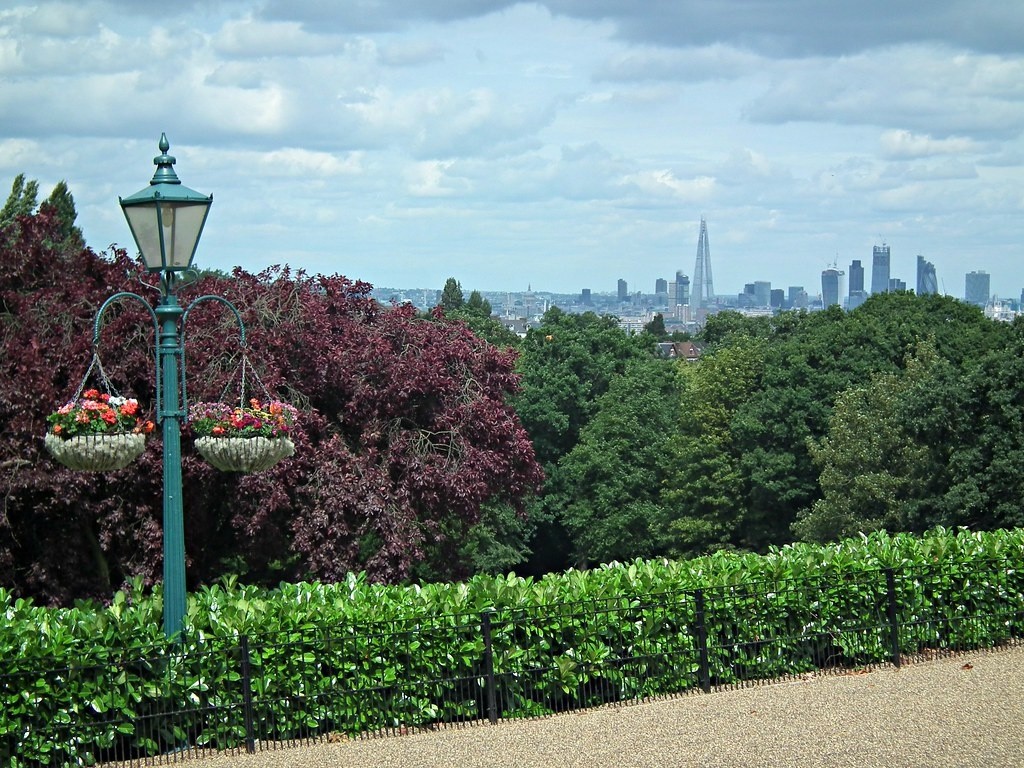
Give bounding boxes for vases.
[45,432,146,471]
[195,436,297,474]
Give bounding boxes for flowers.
[46,389,154,437]
[188,401,297,439]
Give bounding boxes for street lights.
[45,134,297,647]
